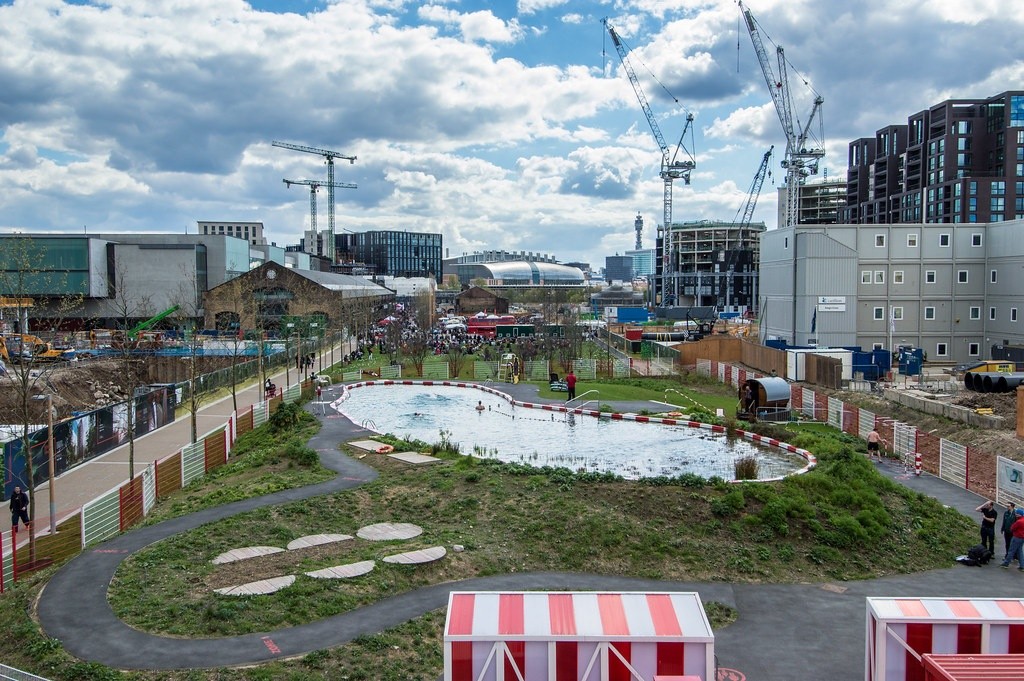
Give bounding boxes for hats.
[1015,508,1024,516]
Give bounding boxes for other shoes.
[990,555,995,559]
[1017,567,1024,571]
[1000,563,1009,568]
[879,460,882,463]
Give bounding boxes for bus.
[466,325,496,337]
[468,315,518,325]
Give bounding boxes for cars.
[519,315,552,325]
[437,311,468,333]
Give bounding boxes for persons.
[741,386,752,410]
[975,501,1024,572]
[566,371,577,401]
[265,378,272,395]
[866,427,883,463]
[9,486,30,535]
[309,371,316,384]
[294,308,607,374]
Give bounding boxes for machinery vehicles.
[88,302,180,346]
[0,297,64,362]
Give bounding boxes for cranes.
[282,178,358,231]
[274,141,357,262]
[733,0,827,227]
[598,14,699,308]
